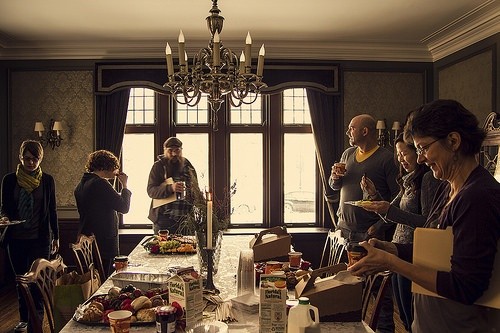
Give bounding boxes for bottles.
[155,305,177,333]
[176,181,186,200]
[286,297,319,333]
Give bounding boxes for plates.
[344,200,386,207]
[0,220,20,226]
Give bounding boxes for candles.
[207,192,212,249]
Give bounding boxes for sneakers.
[15,322,28,332]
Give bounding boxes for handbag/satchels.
[53,270,100,333]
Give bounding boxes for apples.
[108,288,119,298]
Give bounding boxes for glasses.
[415,137,443,155]
[21,156,39,162]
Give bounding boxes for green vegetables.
[159,241,181,254]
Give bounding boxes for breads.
[177,244,193,251]
[295,269,310,277]
[282,262,289,271]
[82,295,163,322]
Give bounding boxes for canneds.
[155,305,177,333]
[265,261,280,274]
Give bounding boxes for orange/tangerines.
[274,280,286,288]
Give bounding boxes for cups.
[287,252,302,268]
[107,310,132,333]
[114,255,128,272]
[334,163,346,176]
[158,230,168,241]
[347,241,368,266]
[238,249,254,271]
[265,261,280,274]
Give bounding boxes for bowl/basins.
[109,272,170,291]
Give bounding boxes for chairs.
[318,229,391,332]
[15,254,68,333]
[69,233,106,281]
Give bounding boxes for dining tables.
[58,234,374,333]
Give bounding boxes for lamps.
[376,120,400,149]
[33,120,63,151]
[162,0,268,131]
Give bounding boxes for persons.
[360,129,441,333]
[73,150,132,282]
[328,114,404,333]
[147,136,205,240]
[340,96,500,333]
[0,138,60,332]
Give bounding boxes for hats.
[164,137,182,147]
